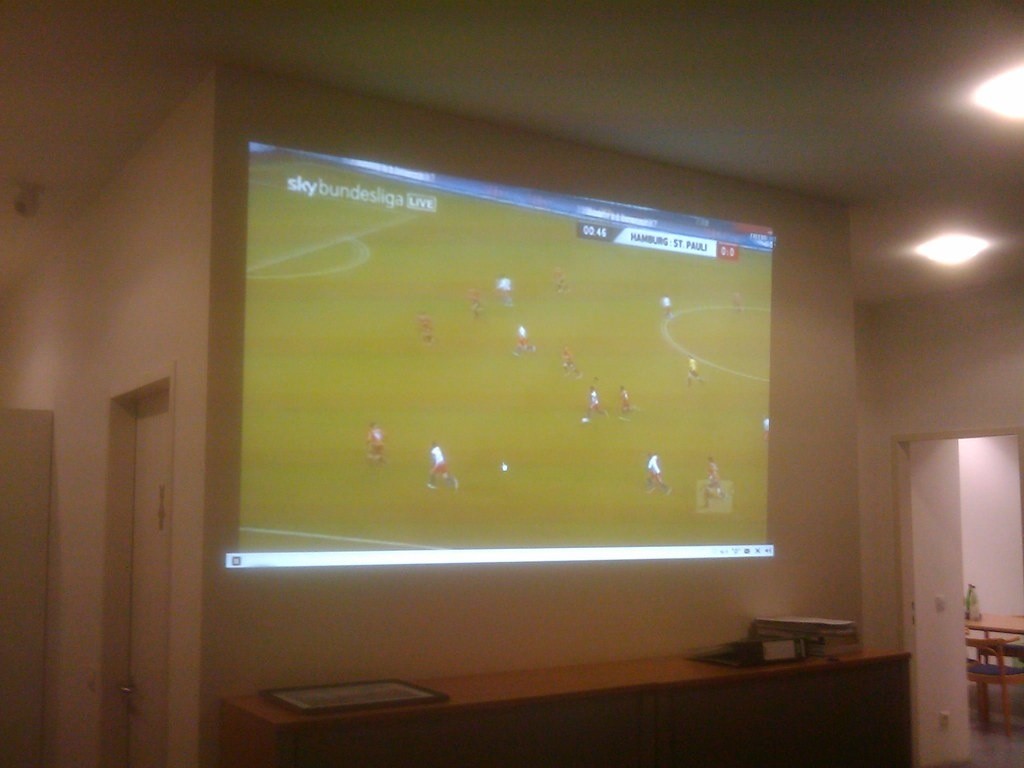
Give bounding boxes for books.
[756,614,861,656]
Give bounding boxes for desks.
[219,647,912,768]
[967,613,1024,664]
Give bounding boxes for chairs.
[966,636,1024,737]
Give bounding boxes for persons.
[687,356,702,385]
[510,324,537,358]
[562,346,581,379]
[581,386,608,423]
[365,422,387,463]
[700,458,726,510]
[733,292,741,308]
[763,416,769,440]
[551,266,573,293]
[493,275,513,307]
[644,450,672,496]
[661,295,674,320]
[618,386,631,419]
[464,288,481,318]
[426,444,460,491]
[418,310,434,345]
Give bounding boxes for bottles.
[966,584,980,622]
